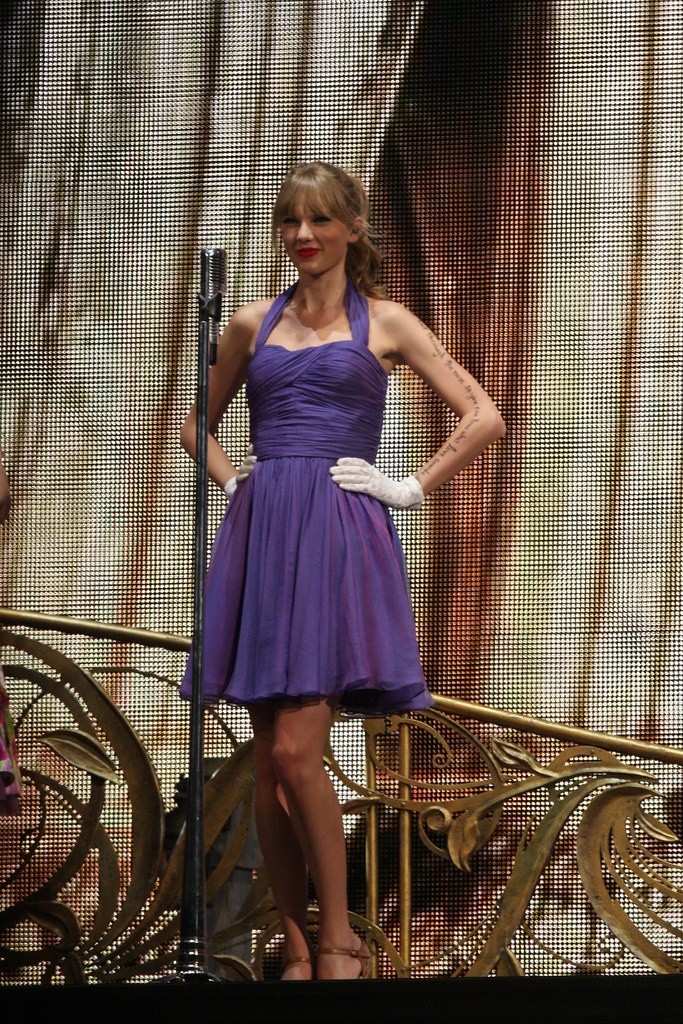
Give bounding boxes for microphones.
[199,248,228,365]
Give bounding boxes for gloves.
[330,456,425,510]
[225,445,258,499]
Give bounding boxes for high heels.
[317,933,373,980]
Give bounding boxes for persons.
[180,161,506,979]
